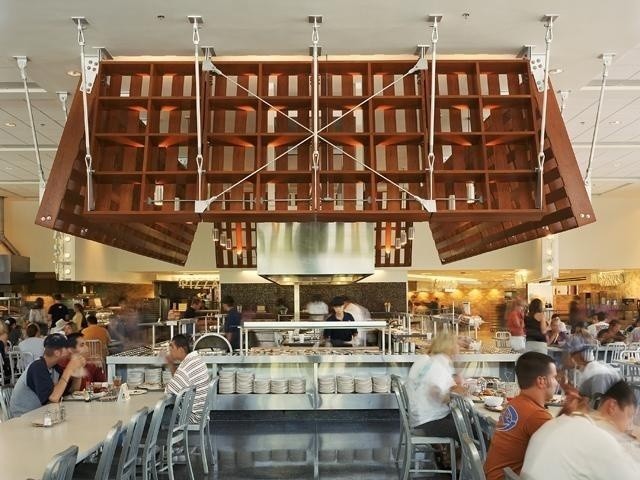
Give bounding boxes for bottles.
[44,405,65,426]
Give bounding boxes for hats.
[43,333,67,346]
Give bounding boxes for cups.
[426,332,432,339]
[288,331,294,341]
[112,376,123,387]
[392,341,416,352]
[467,377,518,401]
[299,334,304,343]
[84,382,114,401]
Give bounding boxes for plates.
[139,386,162,390]
[218,447,392,467]
[484,405,503,411]
[129,389,148,395]
[217,370,390,394]
[127,367,172,386]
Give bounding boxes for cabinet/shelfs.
[86,57,547,221]
[198,409,390,473]
[418,77,598,267]
[108,339,542,407]
[373,190,414,268]
[35,71,205,267]
[209,192,258,268]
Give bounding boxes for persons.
[180,298,207,342]
[323,295,371,348]
[482,351,580,480]
[141,334,211,436]
[404,334,477,480]
[506,294,639,415]
[408,294,438,315]
[1,293,128,418]
[220,296,241,352]
[520,380,638,480]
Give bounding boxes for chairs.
[448,312,638,480]
[2,382,218,479]
[390,379,459,477]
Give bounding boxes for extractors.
[256,221,375,286]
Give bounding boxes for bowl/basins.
[484,396,503,407]
[209,326,221,332]
